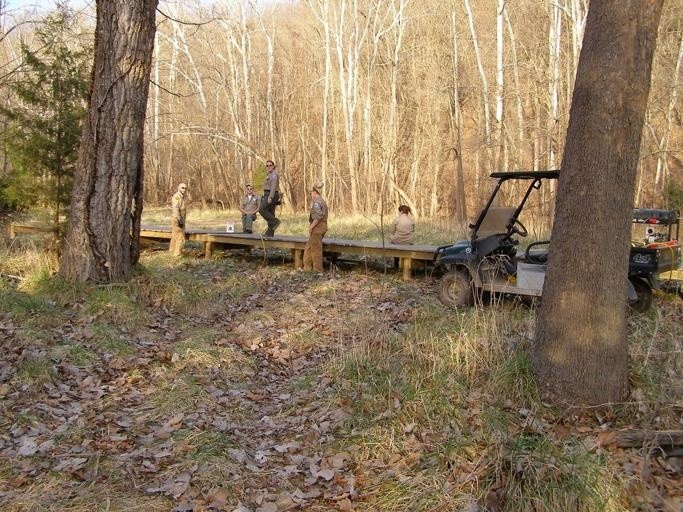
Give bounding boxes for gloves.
[178,219,183,228]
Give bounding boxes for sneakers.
[261,218,281,237]
[395,258,399,268]
[313,272,323,276]
[244,230,252,234]
[173,254,185,258]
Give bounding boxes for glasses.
[247,188,252,190]
[310,193,312,195]
[266,164,272,167]
[182,187,187,189]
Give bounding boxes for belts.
[264,190,278,192]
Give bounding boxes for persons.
[240,183,260,253]
[298,186,329,276]
[388,205,415,268]
[259,160,282,237]
[169,183,192,257]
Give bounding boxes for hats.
[246,183,254,188]
[313,185,321,193]
